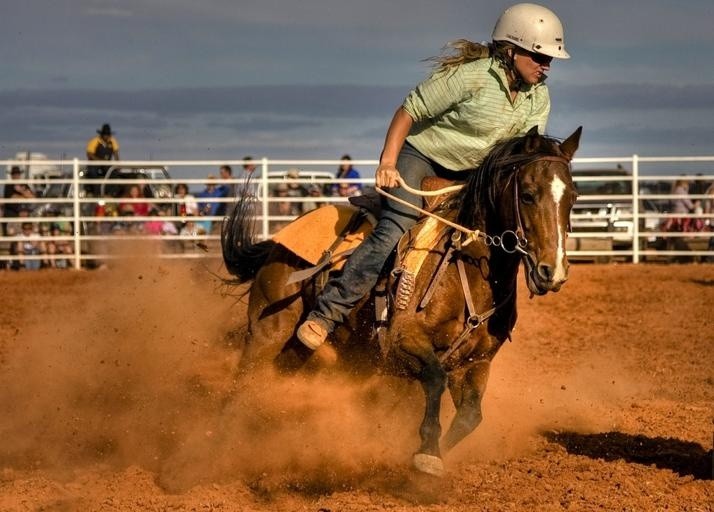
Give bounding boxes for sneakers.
[296,317,329,352]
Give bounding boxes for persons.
[79,122,120,197]
[293,1,570,350]
[671,175,700,217]
[270,154,362,234]
[197,157,256,249]
[1,166,199,272]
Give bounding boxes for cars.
[569,163,671,261]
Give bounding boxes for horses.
[202,123,583,479]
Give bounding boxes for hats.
[96,123,115,136]
[6,167,24,175]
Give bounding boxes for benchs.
[565,237,626,265]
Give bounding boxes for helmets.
[491,1,572,60]
